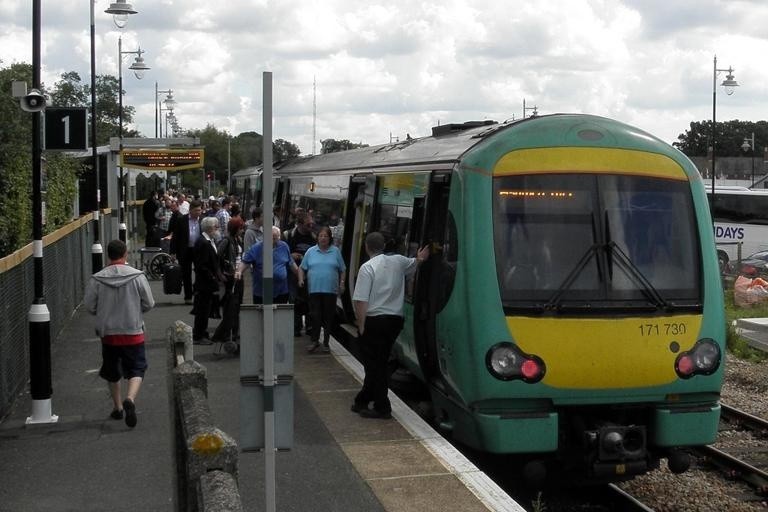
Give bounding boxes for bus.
[698,180,767,272]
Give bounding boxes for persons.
[296,225,348,355]
[348,231,431,420]
[142,184,346,346]
[84,239,156,429]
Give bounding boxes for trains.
[230,114,726,482]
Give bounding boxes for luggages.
[163,261,183,295]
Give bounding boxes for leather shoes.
[350,402,368,412]
[361,407,391,419]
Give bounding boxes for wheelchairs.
[146,241,176,281]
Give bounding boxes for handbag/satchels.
[189,294,221,320]
[288,295,310,314]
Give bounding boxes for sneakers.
[322,344,331,352]
[193,336,213,345]
[123,398,137,428]
[305,341,320,352]
[185,300,193,304]
[110,408,123,420]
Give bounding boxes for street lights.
[117,42,150,139]
[89,0,139,211]
[711,56,739,216]
[156,87,184,139]
[739,132,756,189]
